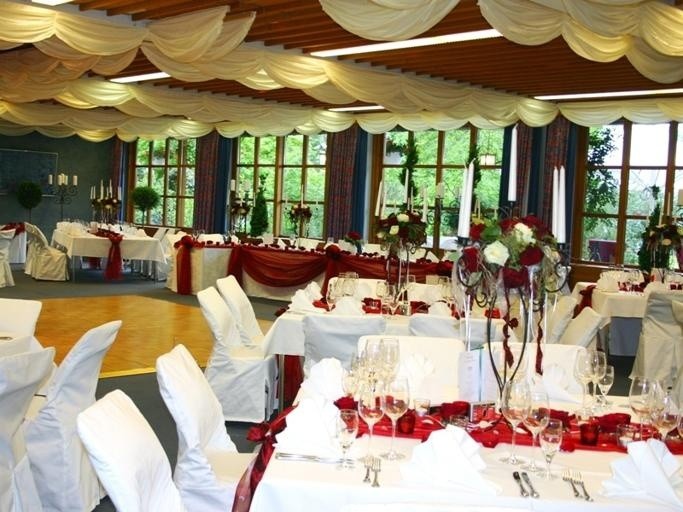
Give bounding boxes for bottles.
[224,230,231,244]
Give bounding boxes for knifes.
[513,472,529,498]
[275,450,353,464]
[521,472,539,497]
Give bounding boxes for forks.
[562,469,580,498]
[370,457,381,487]
[573,471,593,500]
[363,456,373,482]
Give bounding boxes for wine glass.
[500,380,563,477]
[450,414,468,431]
[662,266,683,290]
[607,262,641,296]
[327,272,360,315]
[375,274,416,315]
[193,230,198,242]
[573,348,615,415]
[198,229,205,241]
[339,335,429,460]
[649,389,678,446]
[629,376,656,443]
[437,274,468,317]
[677,408,683,438]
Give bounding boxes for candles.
[645,188,683,227]
[225,179,258,204]
[455,123,565,244]
[87,180,121,201]
[376,170,429,221]
[48,172,77,187]
[283,184,316,208]
